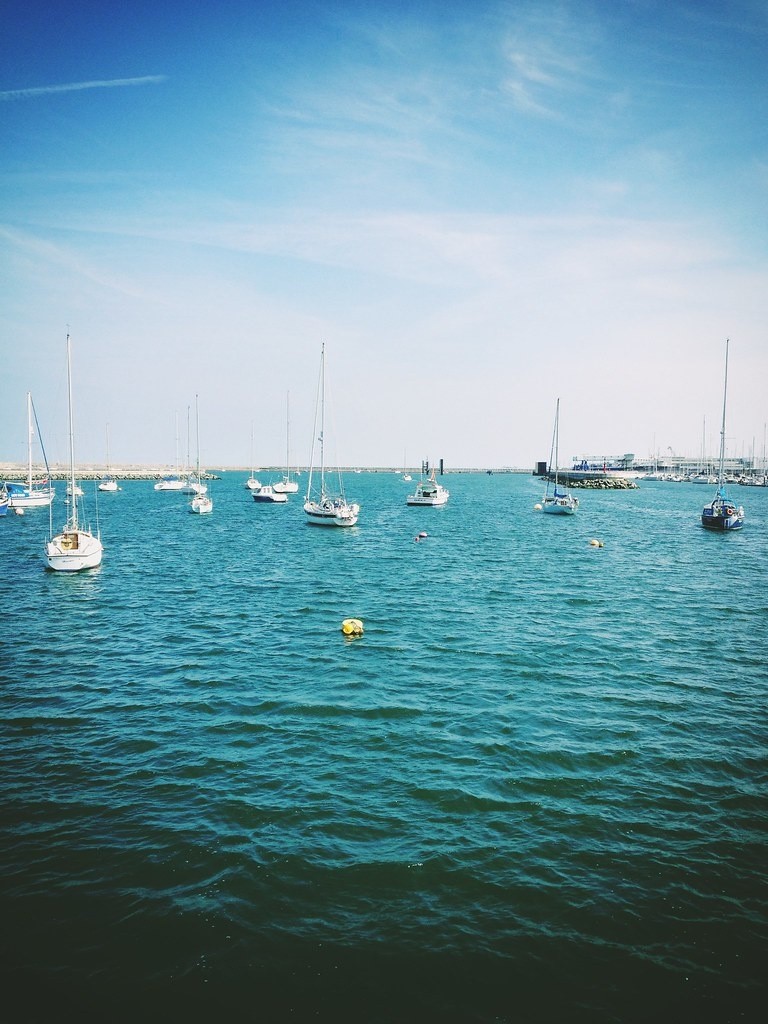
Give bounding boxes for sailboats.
[0,390,55,518]
[41,322,105,572]
[303,342,361,526]
[153,386,213,515]
[245,389,299,503]
[541,397,579,515]
[641,413,768,487]
[407,460,450,505]
[702,336,745,531]
[98,422,123,491]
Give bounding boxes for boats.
[66,474,85,497]
